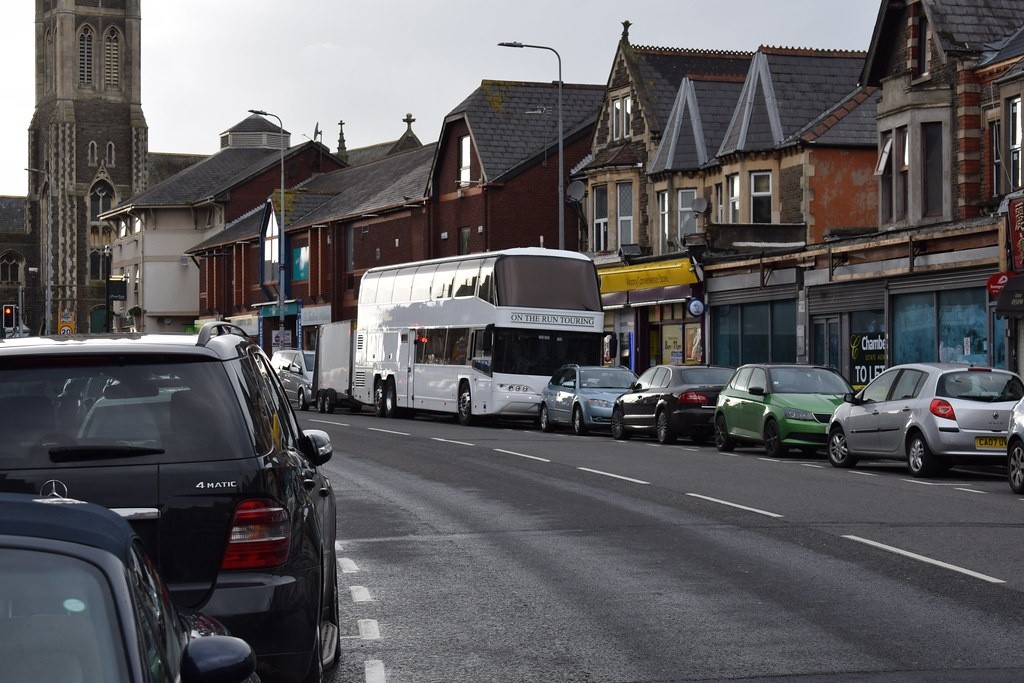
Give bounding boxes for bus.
[352,247,617,426]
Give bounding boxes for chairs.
[161,386,220,454]
[0,396,56,456]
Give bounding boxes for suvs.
[0,322,341,683]
[270,348,316,411]
[537,364,639,435]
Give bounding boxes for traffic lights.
[3,305,15,328]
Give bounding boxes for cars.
[610,364,735,444]
[1007,394,1024,495]
[827,362,1024,478]
[0,490,260,683]
[55,373,192,442]
[713,363,858,457]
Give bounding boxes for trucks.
[311,319,356,414]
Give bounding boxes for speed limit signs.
[60,325,73,335]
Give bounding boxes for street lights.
[497,42,563,249]
[103,245,110,333]
[25,168,52,335]
[248,109,285,350]
